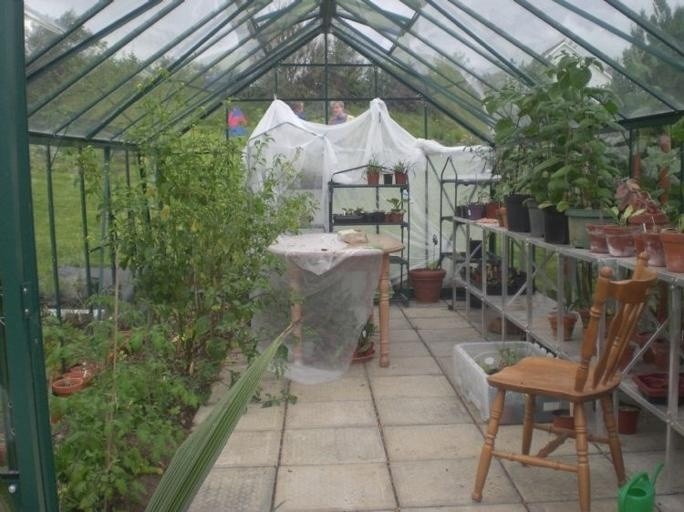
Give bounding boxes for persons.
[328,100,355,125]
[291,99,306,121]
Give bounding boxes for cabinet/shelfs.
[446,214,683,494]
[328,164,410,304]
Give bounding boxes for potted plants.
[408,235,446,305]
[447,53,683,433]
[39,312,88,400]
[334,155,418,226]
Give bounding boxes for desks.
[269,231,406,371]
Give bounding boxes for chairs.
[471,248,658,512]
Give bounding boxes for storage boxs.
[454,338,568,427]
